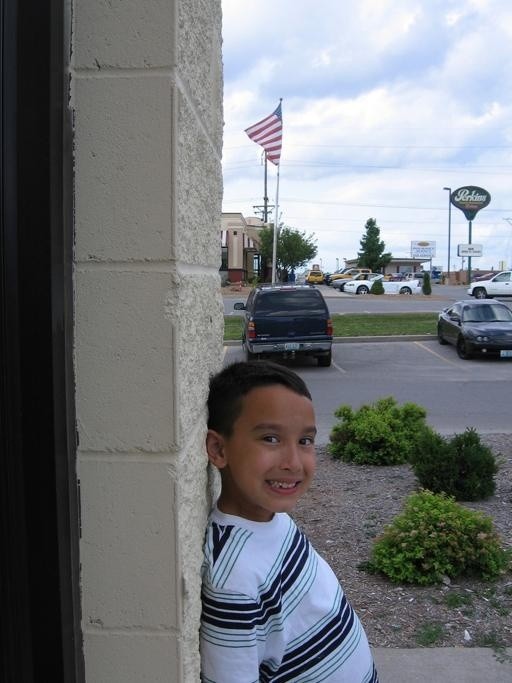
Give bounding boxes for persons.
[196,360,380,682]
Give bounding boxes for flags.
[243,96,284,167]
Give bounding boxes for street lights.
[336,258,339,270]
[443,187,451,285]
[320,258,322,270]
[252,150,280,282]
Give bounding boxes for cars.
[234,282,333,367]
[437,270,512,360]
[304,269,442,296]
